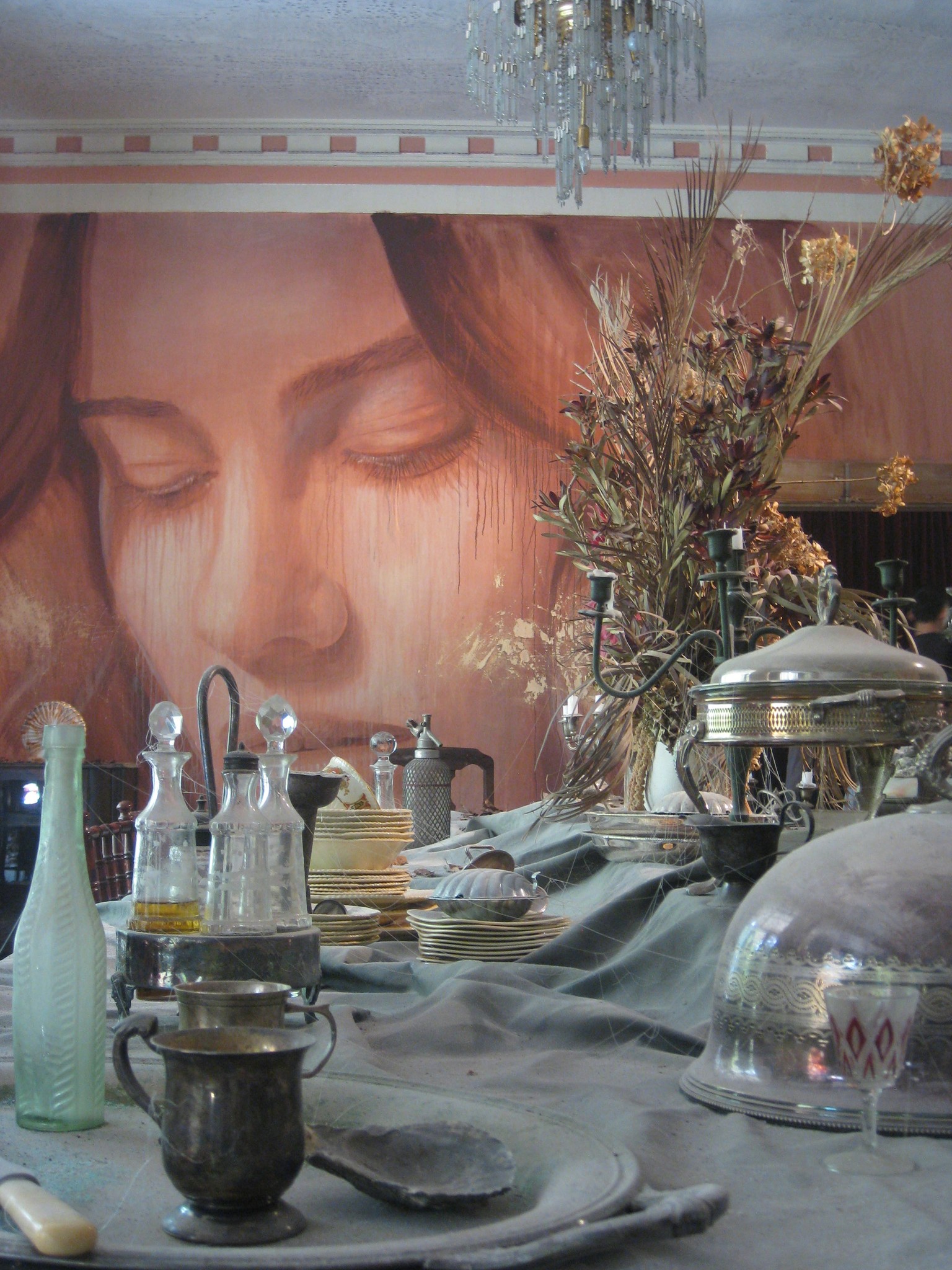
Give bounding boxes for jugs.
[684,800,816,906]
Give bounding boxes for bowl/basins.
[580,812,775,866]
[319,757,381,811]
[309,809,415,870]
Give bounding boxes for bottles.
[370,731,397,809]
[255,694,312,931]
[201,743,277,936]
[127,701,202,934]
[12,725,106,1133]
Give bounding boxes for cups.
[111,1011,318,1245]
[173,981,336,1079]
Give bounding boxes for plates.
[1,1055,640,1268]
[405,909,571,963]
[309,903,381,946]
[307,869,412,905]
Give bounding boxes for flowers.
[512,114,952,834]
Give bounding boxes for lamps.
[466,0,706,211]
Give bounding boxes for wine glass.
[823,984,920,1178]
